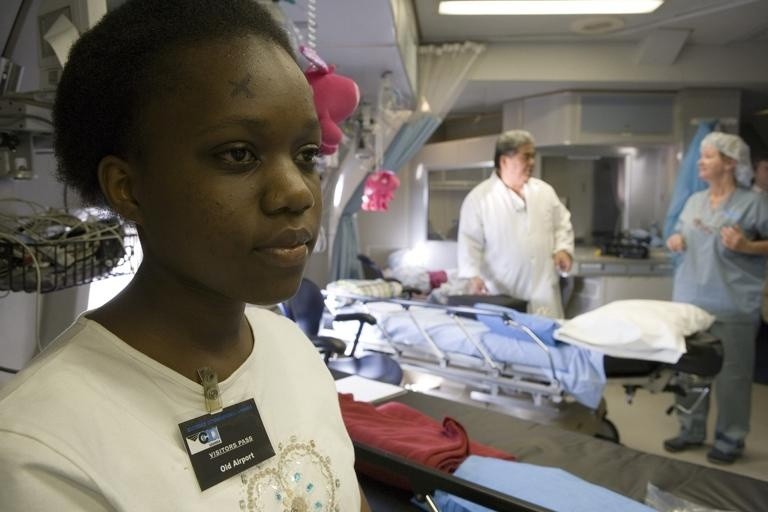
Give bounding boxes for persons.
[1,3,375,511]
[663,129,768,464]
[748,157,768,388]
[456,128,575,319]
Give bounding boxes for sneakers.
[707,448,741,466]
[664,436,703,452]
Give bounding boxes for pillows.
[549,294,718,369]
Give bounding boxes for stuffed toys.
[359,167,401,213]
[301,65,362,152]
